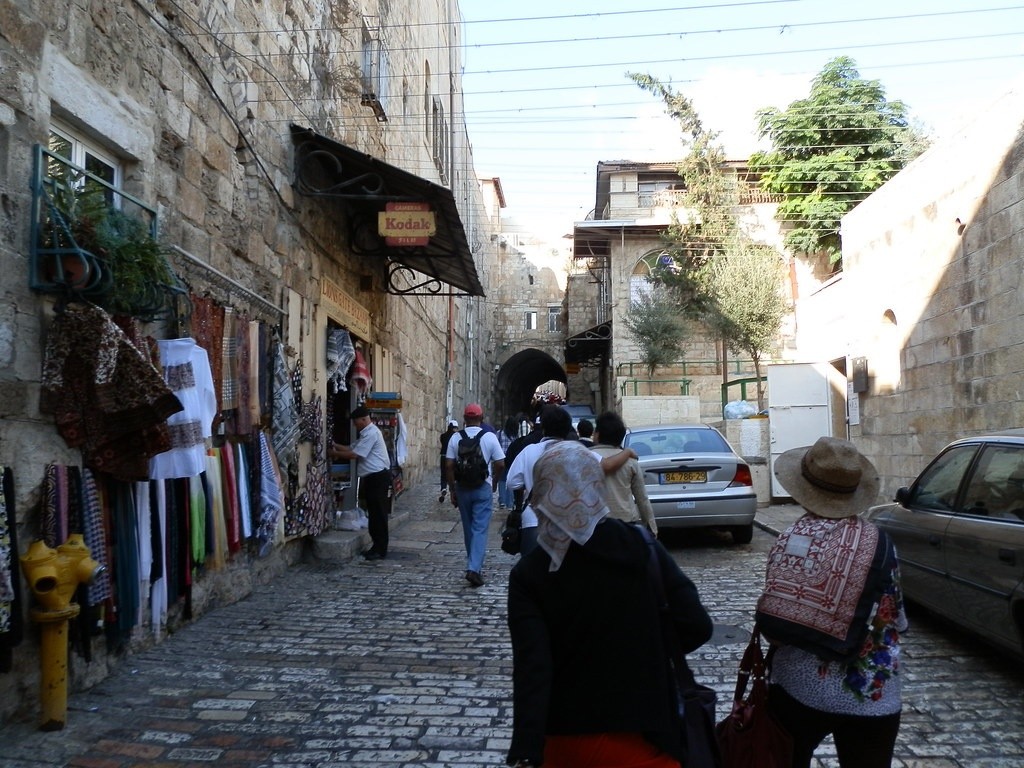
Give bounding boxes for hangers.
[150,316,188,337]
[57,279,91,313]
[23,422,77,466]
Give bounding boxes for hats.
[349,407,368,419]
[464,403,482,418]
[449,420,458,426]
[774,436,881,518]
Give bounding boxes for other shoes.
[500,503,506,509]
[365,545,386,560]
[439,488,447,502]
[466,569,484,586]
[507,507,513,511]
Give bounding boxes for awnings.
[291,123,486,297]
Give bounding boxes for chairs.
[684,441,704,452]
[1000,470,1024,520]
[630,442,653,456]
[330,457,353,490]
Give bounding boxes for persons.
[446,405,506,586]
[482,411,544,511]
[505,405,639,559]
[577,419,594,447]
[328,408,389,561]
[507,440,714,768]
[756,436,908,768]
[439,420,459,503]
[588,411,658,540]
[528,389,567,421]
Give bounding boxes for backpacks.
[453,429,490,489]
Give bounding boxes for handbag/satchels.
[501,508,523,554]
[674,683,718,768]
[713,622,794,768]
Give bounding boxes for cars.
[567,404,598,439]
[859,426,1023,662]
[619,427,757,545]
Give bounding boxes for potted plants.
[36,172,190,320]
